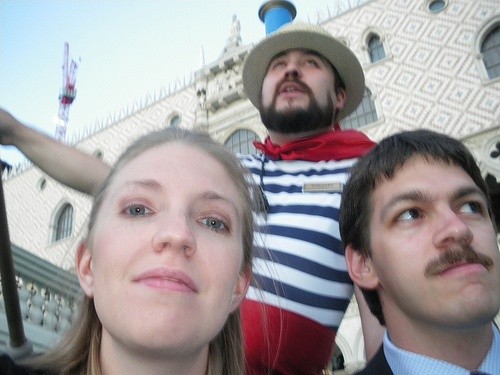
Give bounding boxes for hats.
[241,21,365,121]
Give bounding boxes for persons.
[0,127,254,375]
[1,24,386,375]
[337,127,499,375]
